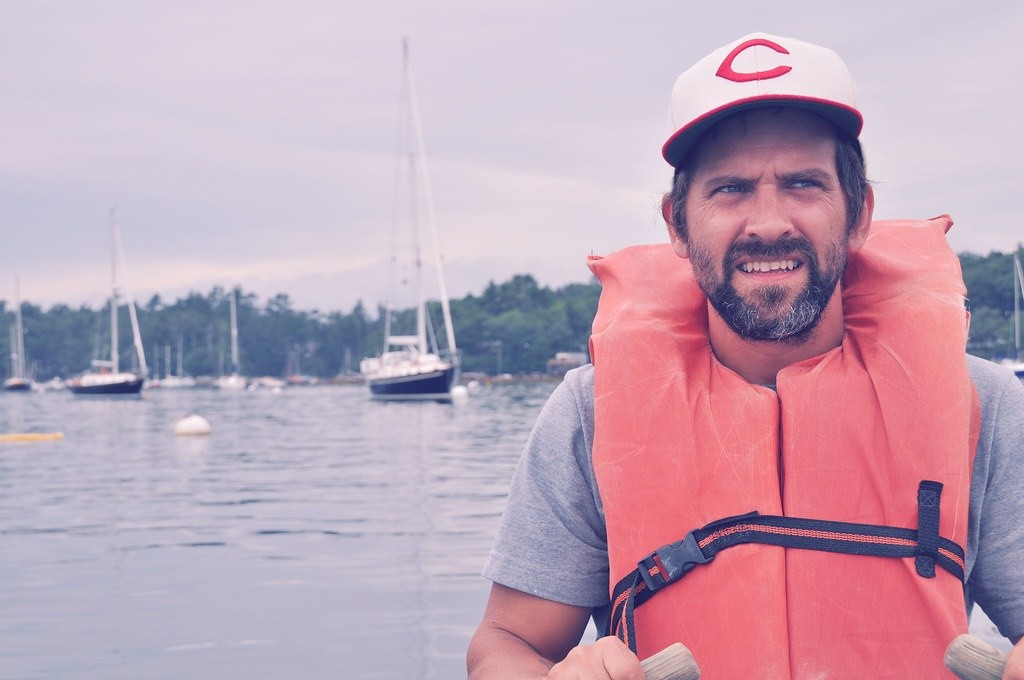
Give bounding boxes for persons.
[466,30,1024,680]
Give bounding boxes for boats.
[331,343,361,385]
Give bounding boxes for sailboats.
[1,275,68,392]
[359,37,462,403]
[142,286,318,389]
[67,207,152,394]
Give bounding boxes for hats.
[662,32,864,168]
